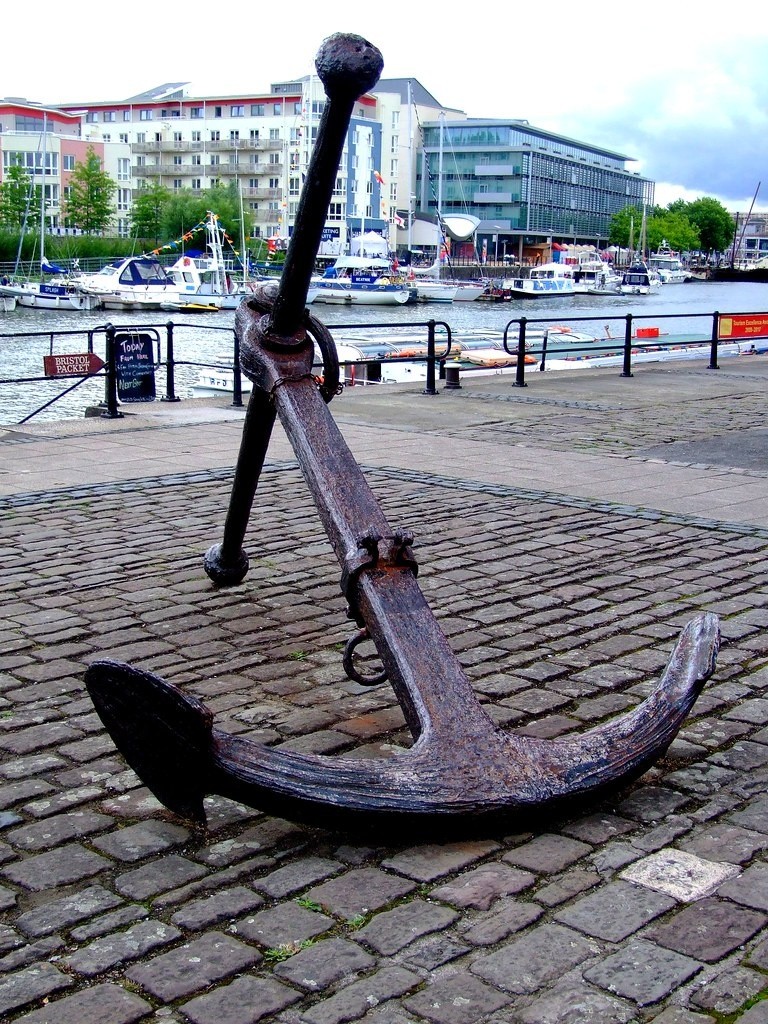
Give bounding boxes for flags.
[396,215,405,227]
[374,170,384,185]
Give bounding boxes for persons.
[484,284,511,296]
[535,250,542,266]
[2,274,12,286]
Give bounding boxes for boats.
[574,260,622,292]
[158,301,219,313]
[683,262,712,282]
[620,201,663,295]
[188,349,255,398]
[51,256,182,312]
[589,287,623,296]
[650,241,688,284]
[501,263,577,296]
[479,288,511,302]
[163,251,255,309]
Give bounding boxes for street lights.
[493,226,502,262]
[595,234,601,260]
[548,229,554,262]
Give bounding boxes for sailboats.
[228,61,486,305]
[0,112,102,310]
